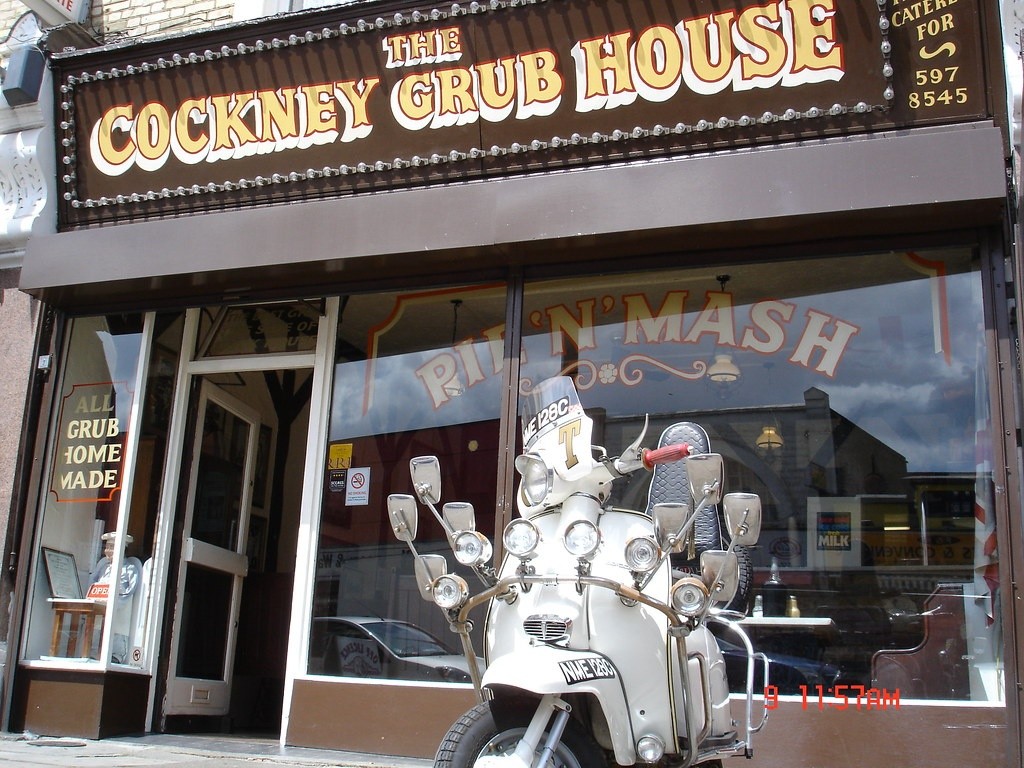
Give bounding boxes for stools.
[46,598,106,660]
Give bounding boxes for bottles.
[788,516,801,567]
[752,595,763,618]
[785,599,800,617]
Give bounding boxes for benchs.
[870,582,974,700]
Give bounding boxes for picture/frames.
[233,510,268,566]
[320,448,357,531]
[41,546,84,599]
[229,417,273,509]
[141,340,177,439]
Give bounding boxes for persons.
[79,532,139,664]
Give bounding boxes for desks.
[735,616,840,638]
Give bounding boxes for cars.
[307,614,489,685]
[712,631,872,700]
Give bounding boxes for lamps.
[702,274,745,402]
[753,409,786,460]
[442,298,466,400]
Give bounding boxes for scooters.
[385,377,770,768]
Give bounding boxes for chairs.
[646,422,724,567]
